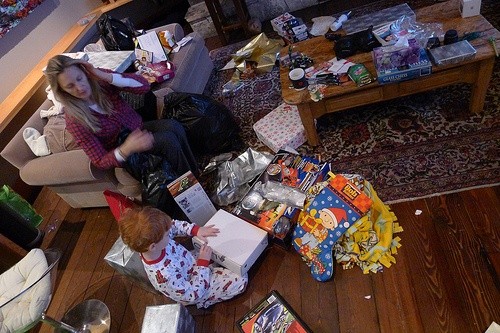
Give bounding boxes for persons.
[119,207,248,310]
[47,55,198,210]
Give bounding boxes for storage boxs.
[104,235,160,296]
[140,303,195,333]
[428,39,476,67]
[191,209,268,276]
[375,47,432,84]
[232,149,330,252]
[253,102,317,154]
[459,0,481,18]
[270,12,309,42]
[167,170,217,227]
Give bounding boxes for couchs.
[0,22,213,208]
[184,0,328,39]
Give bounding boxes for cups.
[267,162,283,182]
[289,68,308,91]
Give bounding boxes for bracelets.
[117,147,128,161]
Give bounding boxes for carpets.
[202,0,500,204]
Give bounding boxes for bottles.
[427,37,440,50]
[444,29,458,45]
[330,11,353,32]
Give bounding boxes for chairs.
[0,247,111,333]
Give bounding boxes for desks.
[280,0,500,146]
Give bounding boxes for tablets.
[236,288,314,333]
